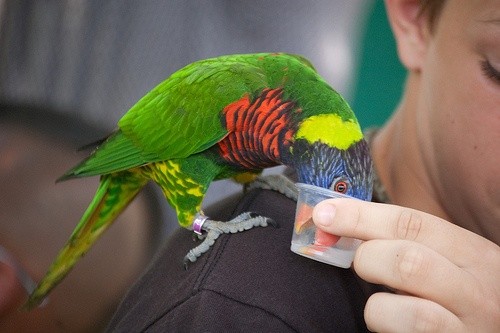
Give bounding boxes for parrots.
[21,52,371,311]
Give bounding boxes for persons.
[107,1,500,333]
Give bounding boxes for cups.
[290,182,362,269]
